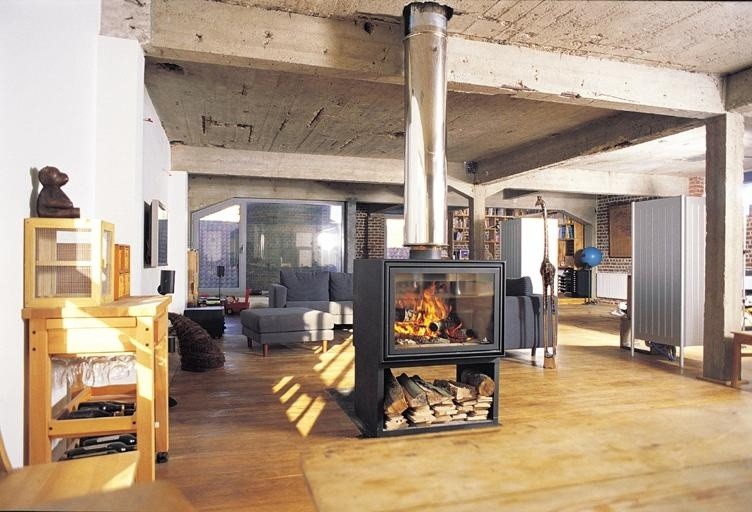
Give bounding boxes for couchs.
[268,282,558,357]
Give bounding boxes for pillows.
[329,271,353,301]
[280,269,330,301]
[506,276,533,296]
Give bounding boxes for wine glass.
[47,355,137,391]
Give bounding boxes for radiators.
[596,272,631,300]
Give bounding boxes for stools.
[239,306,335,357]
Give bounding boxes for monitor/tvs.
[151,199,168,267]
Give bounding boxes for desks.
[730,331,752,387]
[22,293,173,484]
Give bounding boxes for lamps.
[217,264,225,306]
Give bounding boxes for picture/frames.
[607,203,631,258]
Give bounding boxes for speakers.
[217,266,224,277]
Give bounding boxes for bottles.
[59,401,137,462]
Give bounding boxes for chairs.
[0,427,140,512]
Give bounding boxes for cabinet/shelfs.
[24,216,115,308]
[188,251,199,307]
[185,305,226,340]
[56,242,130,301]
[448,210,584,268]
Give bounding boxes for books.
[197,296,221,307]
